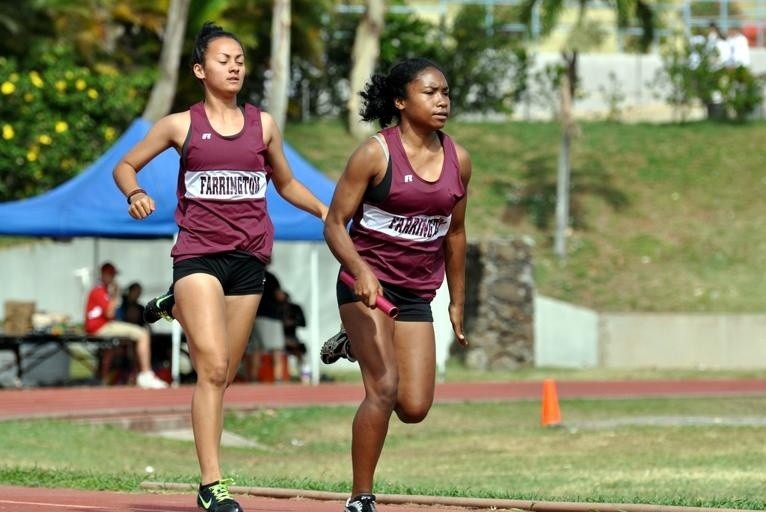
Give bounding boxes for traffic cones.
[540,378,565,430]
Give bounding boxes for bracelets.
[127,190,147,202]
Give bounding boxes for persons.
[687,13,758,70]
[82,264,170,389]
[237,272,309,385]
[111,20,328,511]
[320,59,470,511]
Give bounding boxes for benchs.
[1,329,198,386]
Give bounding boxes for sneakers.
[198,481,240,512]
[321,321,356,365]
[144,288,176,323]
[343,494,376,512]
[136,371,168,391]
